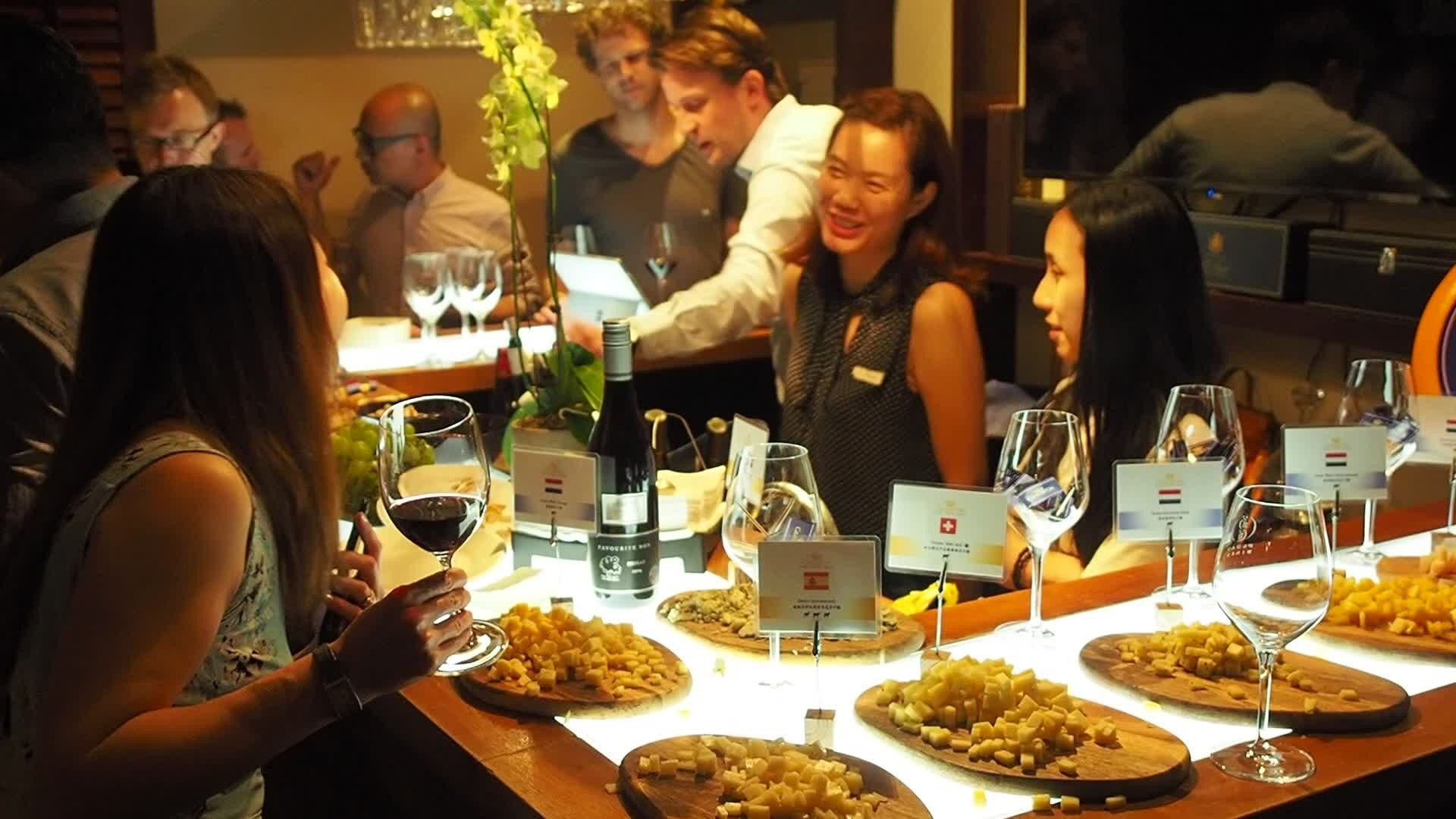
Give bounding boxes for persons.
[1019,1,1456,212]
[0,0,1264,819]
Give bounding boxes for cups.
[549,224,598,296]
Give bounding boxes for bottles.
[589,317,658,602]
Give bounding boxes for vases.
[501,390,593,472]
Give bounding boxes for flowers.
[431,0,605,428]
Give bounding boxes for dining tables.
[329,457,1456,819]
[339,313,774,398]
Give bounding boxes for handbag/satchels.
[1212,366,1278,544]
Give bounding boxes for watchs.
[312,642,367,719]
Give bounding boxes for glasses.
[359,132,416,154]
[130,130,210,152]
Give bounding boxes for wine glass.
[1335,359,1416,566]
[402,252,502,346]
[1210,485,1332,783]
[642,222,679,302]
[993,409,1091,652]
[721,442,825,689]
[1152,384,1246,609]
[377,394,510,676]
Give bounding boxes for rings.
[361,590,375,609]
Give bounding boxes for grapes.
[328,417,436,521]
